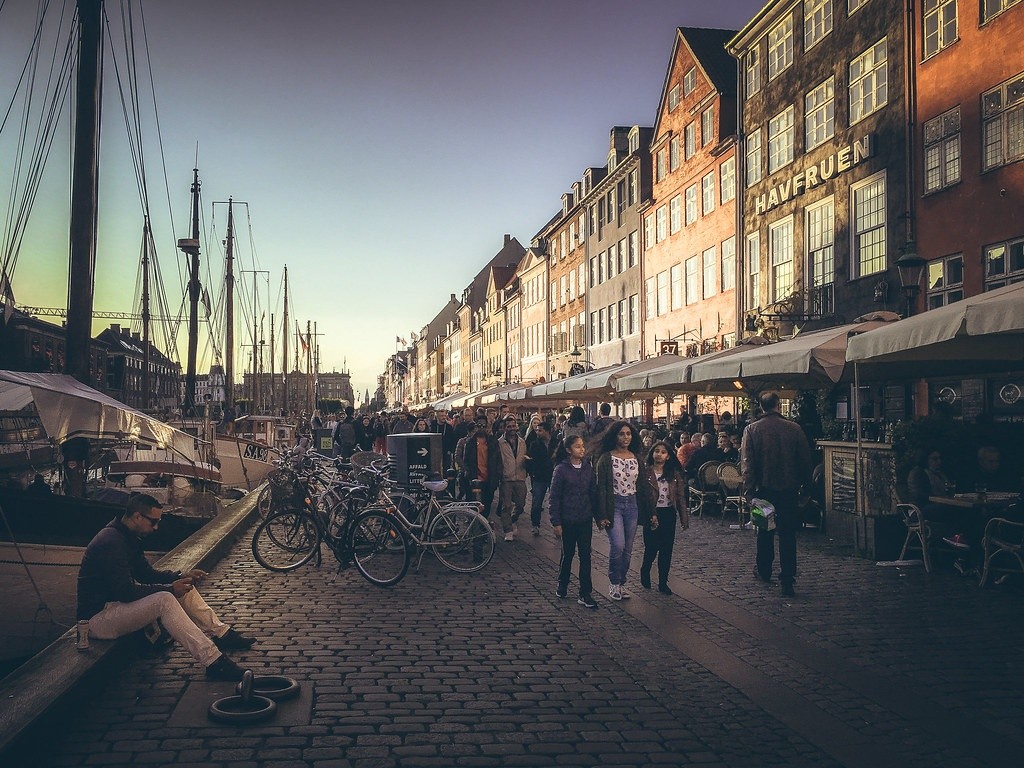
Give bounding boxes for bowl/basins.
[954,493,963,497]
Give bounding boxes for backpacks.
[340,420,356,447]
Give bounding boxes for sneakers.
[617,583,630,599]
[556,585,567,597]
[578,593,598,607]
[608,581,622,600]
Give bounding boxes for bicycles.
[252,444,496,587]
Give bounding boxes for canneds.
[76,619,89,650]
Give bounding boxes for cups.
[976,487,987,503]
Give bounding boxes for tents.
[375,281,1024,519]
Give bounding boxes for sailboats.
[0,1,321,549]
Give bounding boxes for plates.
[985,497,1009,501]
[985,493,1004,498]
[1002,493,1021,498]
[985,491,1002,494]
[963,492,978,498]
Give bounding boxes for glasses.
[926,456,941,461]
[475,423,487,428]
[138,513,161,527]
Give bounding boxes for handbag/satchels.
[750,496,778,531]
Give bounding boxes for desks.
[929,492,1019,577]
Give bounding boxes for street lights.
[895,232,927,439]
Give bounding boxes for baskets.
[351,450,388,483]
[268,467,302,514]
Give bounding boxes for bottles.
[833,417,906,445]
[943,480,957,499]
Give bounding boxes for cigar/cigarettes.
[200,572,209,577]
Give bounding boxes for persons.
[309,394,1024,608]
[76,493,258,682]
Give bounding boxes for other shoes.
[531,526,540,536]
[472,550,482,564]
[205,653,247,682]
[504,531,514,542]
[211,628,257,650]
[943,533,970,549]
[641,567,652,589]
[781,581,794,597]
[511,522,518,535]
[658,580,672,595]
[753,565,767,583]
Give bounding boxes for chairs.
[890,486,949,571]
[978,517,1024,589]
[717,461,751,530]
[688,461,724,520]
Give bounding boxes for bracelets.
[178,573,183,578]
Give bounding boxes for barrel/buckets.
[350,451,387,485]
